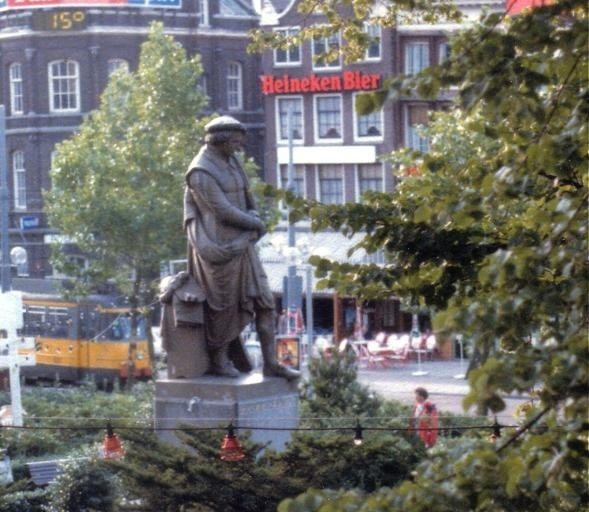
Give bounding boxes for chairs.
[315,329,437,370]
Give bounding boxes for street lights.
[0,244,28,440]
[271,236,312,335]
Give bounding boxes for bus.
[3,295,155,392]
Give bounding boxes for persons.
[183,115,302,378]
[409,386,439,448]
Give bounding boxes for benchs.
[24,457,93,488]
